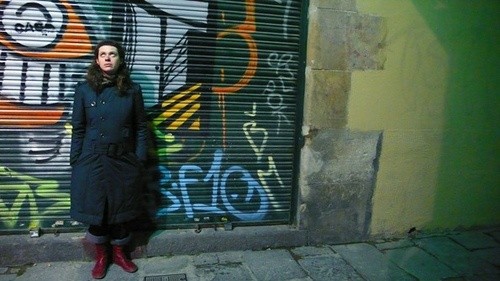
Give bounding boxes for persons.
[68,38,153,279]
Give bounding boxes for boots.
[111,244,138,271]
[92,242,111,279]
